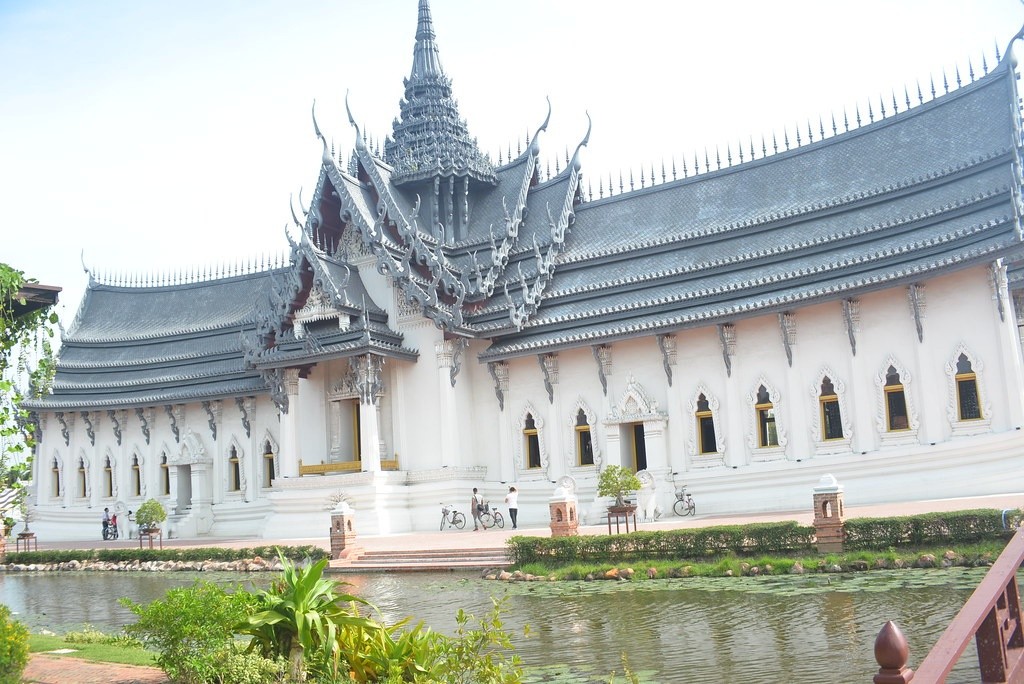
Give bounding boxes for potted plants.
[136,499,167,533]
[597,465,642,512]
[17,508,37,537]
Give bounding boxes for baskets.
[675,492,683,500]
[442,508,450,514]
[483,504,489,513]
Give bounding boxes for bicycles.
[101,522,117,540]
[673,484,696,517]
[439,502,466,530]
[481,500,505,529]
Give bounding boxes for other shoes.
[512,528,517,530]
[473,529,478,531]
[512,526,515,528]
[484,526,487,530]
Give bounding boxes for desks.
[608,512,636,535]
[17,537,38,553]
[139,533,162,549]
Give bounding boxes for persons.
[505,486,519,530]
[102,507,111,540]
[111,512,118,539]
[471,488,487,531]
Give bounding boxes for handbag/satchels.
[477,504,483,511]
